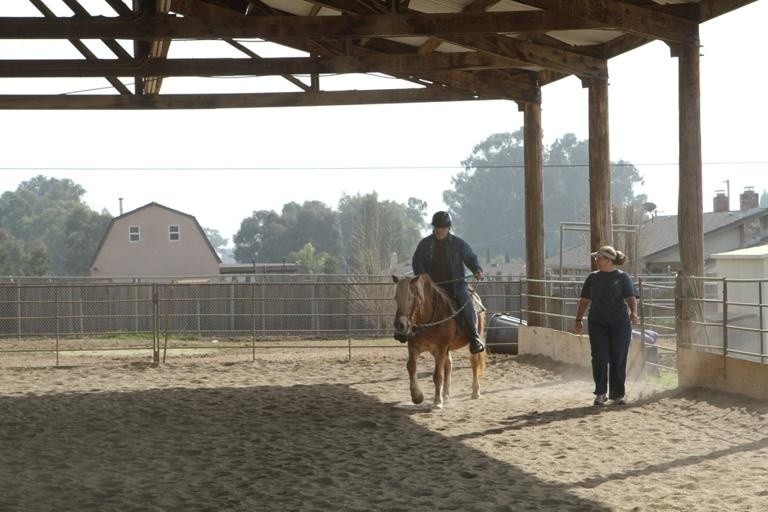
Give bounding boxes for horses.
[391,271,487,414]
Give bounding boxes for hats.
[590,247,617,260]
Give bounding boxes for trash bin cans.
[486,313,528,353]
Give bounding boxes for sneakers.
[469,336,483,353]
[394,331,407,343]
[615,397,625,404]
[594,393,608,405]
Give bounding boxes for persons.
[574,246,640,407]
[412,210,485,354]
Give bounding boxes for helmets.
[430,211,452,228]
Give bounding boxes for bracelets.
[575,319,583,322]
